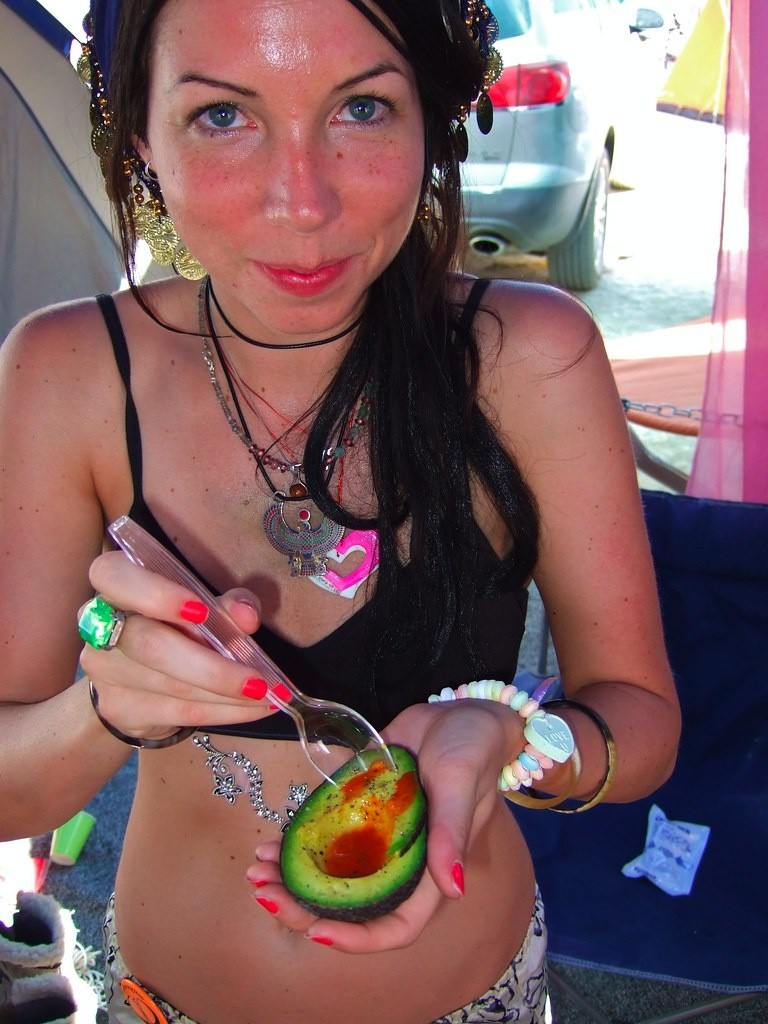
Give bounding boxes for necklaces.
[195,275,381,602]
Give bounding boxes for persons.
[1,2,682,1024]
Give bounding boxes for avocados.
[281,745,426,923]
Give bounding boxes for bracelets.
[501,744,584,810]
[89,677,201,749]
[426,679,576,793]
[538,698,616,813]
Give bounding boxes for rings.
[77,598,140,650]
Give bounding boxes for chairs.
[507,497,767,1023]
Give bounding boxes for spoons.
[110,515,396,791]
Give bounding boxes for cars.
[417,1,666,293]
